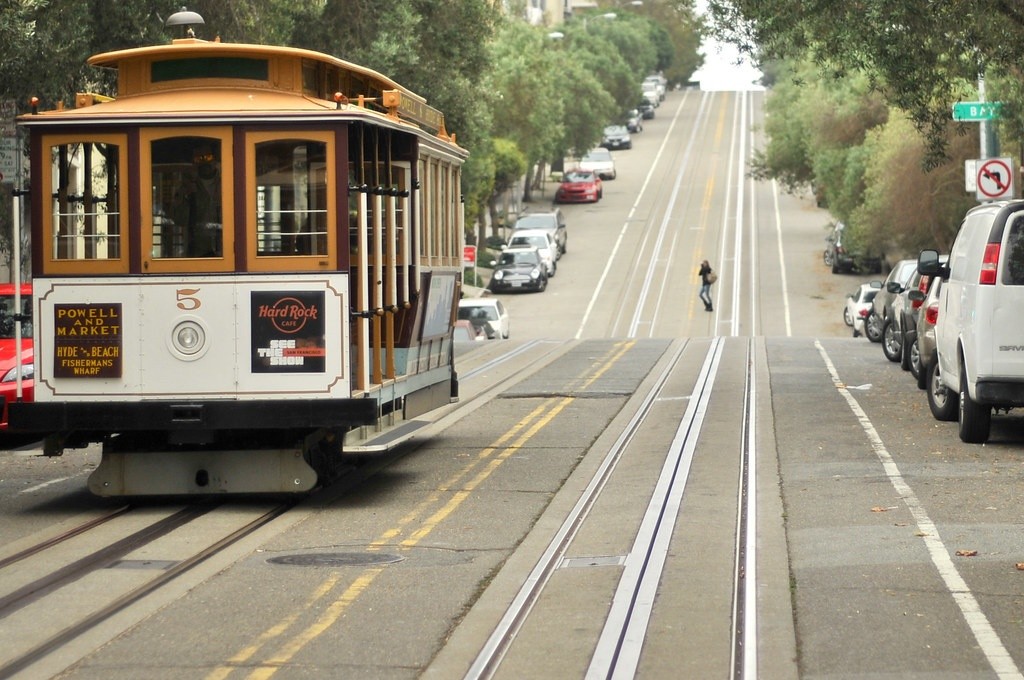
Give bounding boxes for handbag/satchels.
[706,269,717,283]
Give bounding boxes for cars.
[501,229,558,277]
[620,73,667,133]
[457,298,510,340]
[864,255,949,392]
[599,125,632,150]
[555,169,604,203]
[0,282,33,431]
[579,147,617,181]
[823,220,886,275]
[489,249,548,294]
[843,283,882,338]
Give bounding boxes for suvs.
[513,206,567,256]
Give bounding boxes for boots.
[704,303,712,311]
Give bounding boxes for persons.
[170,143,220,257]
[699,260,713,311]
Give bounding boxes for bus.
[15,11,469,496]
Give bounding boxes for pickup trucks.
[452,318,488,341]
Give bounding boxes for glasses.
[193,150,213,161]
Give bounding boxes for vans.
[917,200,1023,444]
